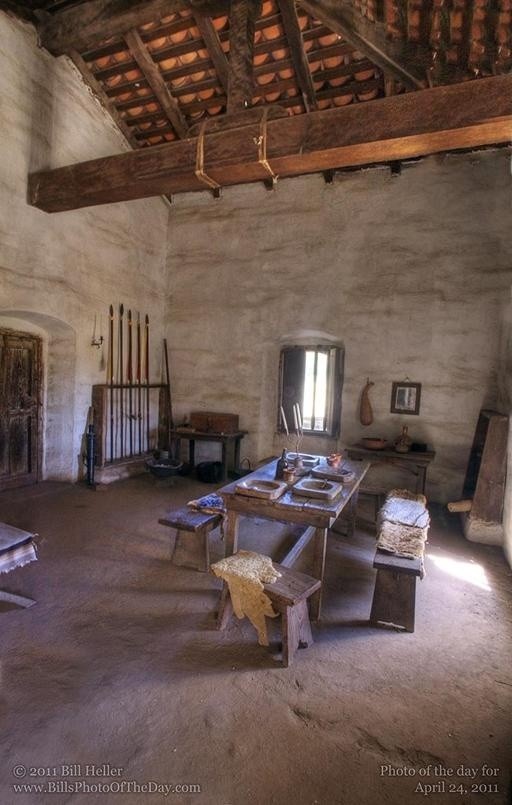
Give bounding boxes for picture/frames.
[390,381,421,415]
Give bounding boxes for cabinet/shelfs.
[461,408,508,545]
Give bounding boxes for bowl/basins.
[145,459,183,478]
[361,439,389,451]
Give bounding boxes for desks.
[170,428,246,481]
[0,522,37,609]
[345,443,435,495]
[217,456,371,620]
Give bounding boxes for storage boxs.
[191,412,238,433]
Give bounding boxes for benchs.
[158,493,224,571]
[370,489,427,633]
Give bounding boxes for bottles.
[275,448,289,479]
[393,424,413,453]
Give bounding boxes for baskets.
[392,426,414,454]
[232,458,254,481]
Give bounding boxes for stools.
[213,551,321,667]
